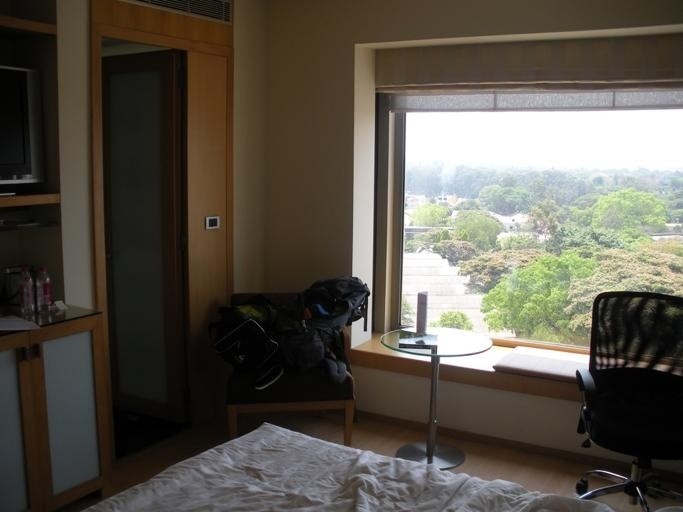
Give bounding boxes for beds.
[78,421,615,512]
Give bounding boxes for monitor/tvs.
[0,66,43,196]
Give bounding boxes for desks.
[379,325,493,469]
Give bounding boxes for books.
[397,330,437,349]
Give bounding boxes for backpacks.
[206,271,370,392]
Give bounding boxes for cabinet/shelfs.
[0,312,113,512]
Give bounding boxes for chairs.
[226,292,356,446]
[574,290,682,512]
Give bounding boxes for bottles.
[18,266,34,315]
[35,266,51,313]
